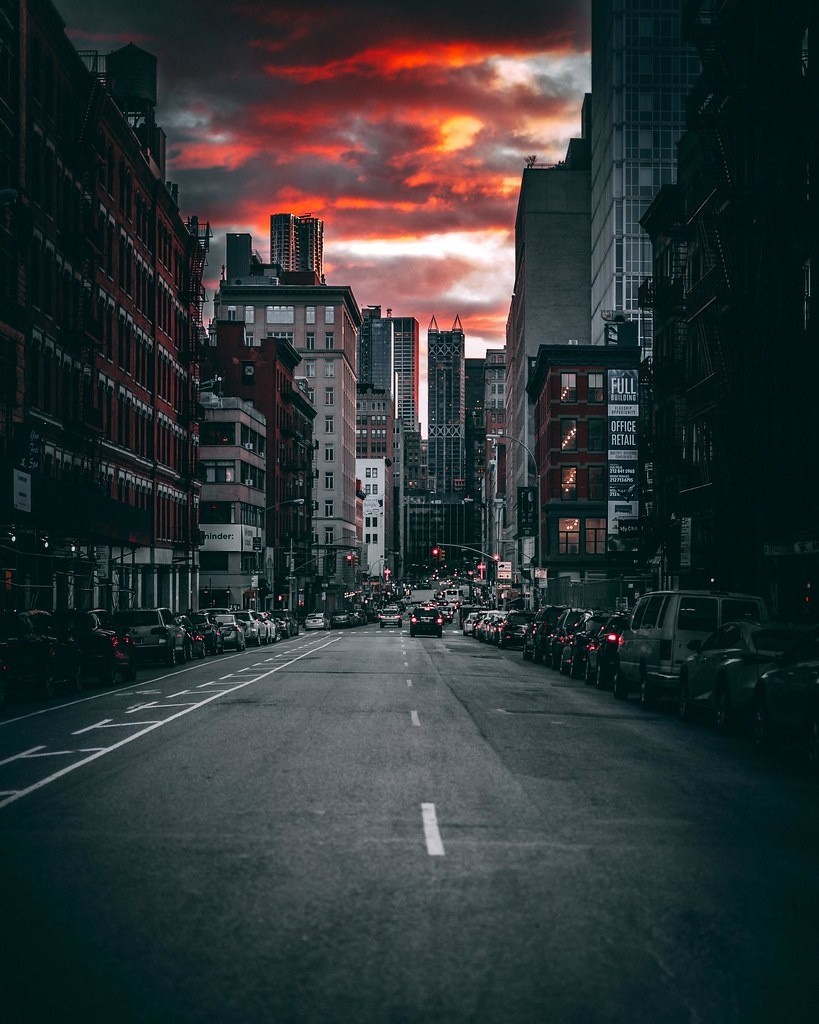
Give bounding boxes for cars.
[0,580,818,778]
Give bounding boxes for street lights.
[369,558,388,592]
[485,432,543,608]
[253,498,304,611]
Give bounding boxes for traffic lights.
[346,553,358,567]
[432,548,445,562]
[277,594,283,602]
[799,582,814,609]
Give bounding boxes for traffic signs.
[496,561,512,572]
[496,583,512,590]
